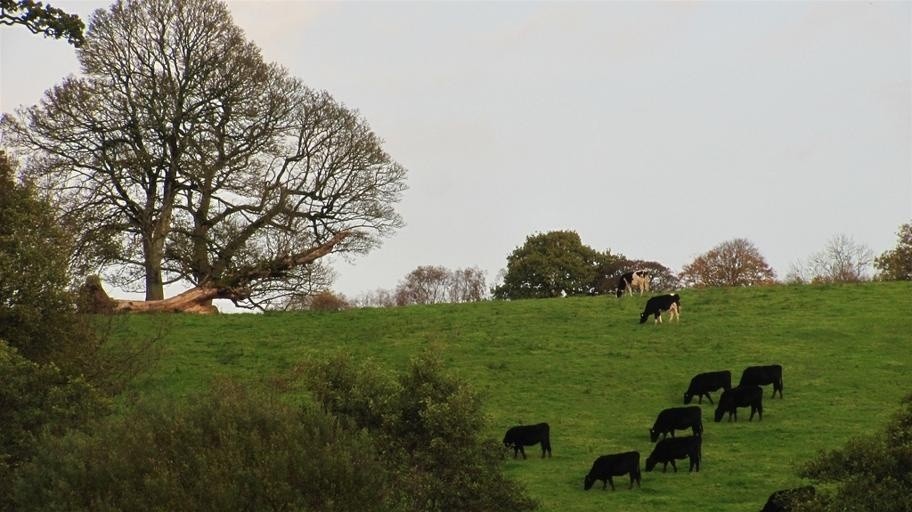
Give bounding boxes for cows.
[645,407,704,473]
[504,423,551,460]
[612,269,651,299]
[584,452,640,492]
[760,485,815,511]
[640,293,682,327]
[684,365,783,424]
